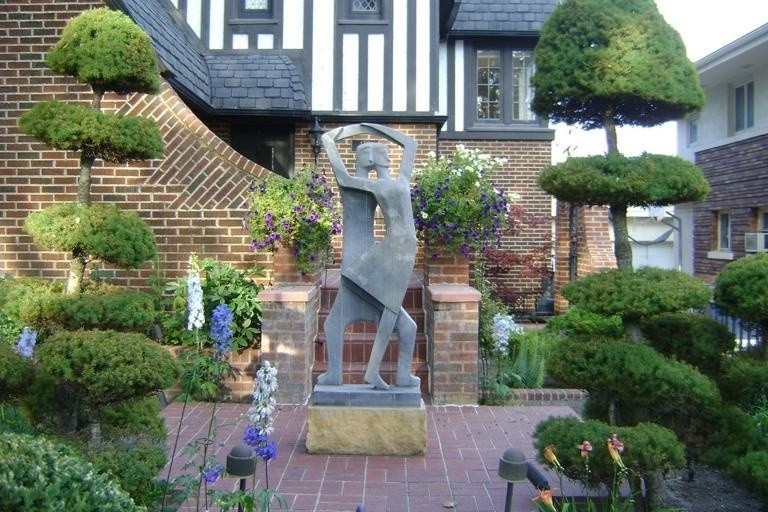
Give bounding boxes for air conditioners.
[743,232,767,254]
